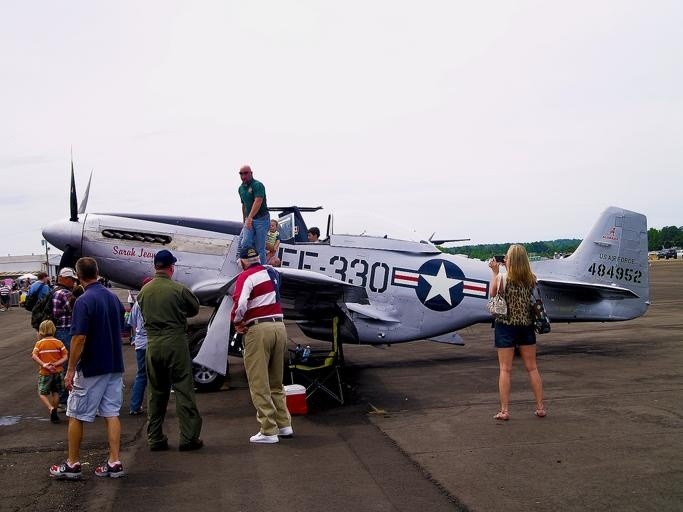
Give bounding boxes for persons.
[488,244,551,421]
[49,256,125,479]
[236,165,271,269]
[127,276,155,415]
[136,248,204,451]
[306,226,321,243]
[230,246,294,444]
[265,218,281,267]
[31,318,70,423]
[0,266,85,415]
[127,290,136,307]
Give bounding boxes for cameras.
[494,256,505,263]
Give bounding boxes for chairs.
[286,316,348,406]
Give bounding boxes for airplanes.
[41,146,652,390]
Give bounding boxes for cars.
[676,250,683,257]
[650,251,660,255]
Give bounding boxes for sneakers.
[95,459,124,478]
[150,440,168,451]
[179,438,203,451]
[50,408,57,422]
[129,406,144,415]
[58,403,67,409]
[278,425,293,435]
[250,431,279,444]
[49,459,82,479]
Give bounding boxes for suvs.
[657,248,677,260]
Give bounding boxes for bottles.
[302,345,310,360]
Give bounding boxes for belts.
[246,317,283,326]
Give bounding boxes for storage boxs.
[285,384,308,415]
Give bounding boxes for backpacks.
[30,285,70,332]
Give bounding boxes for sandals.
[535,408,546,416]
[493,410,510,420]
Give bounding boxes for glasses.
[239,171,251,175]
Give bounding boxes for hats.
[154,250,177,268]
[58,267,78,280]
[239,247,259,259]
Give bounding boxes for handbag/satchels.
[487,272,508,316]
[24,284,45,312]
[531,288,551,334]
